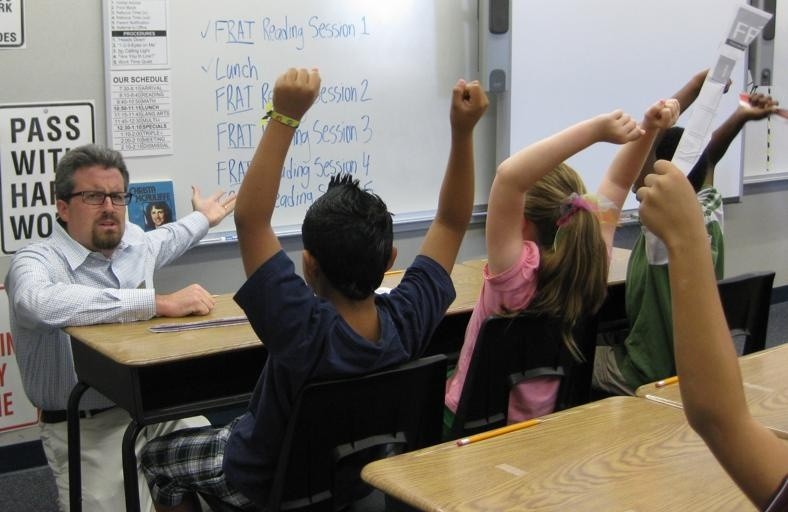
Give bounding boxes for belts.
[41,405,115,424]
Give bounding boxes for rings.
[222,204,229,213]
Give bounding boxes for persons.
[592,64,781,397]
[4,139,239,511]
[635,154,787,510]
[440,97,682,427]
[142,63,488,512]
[146,200,173,231]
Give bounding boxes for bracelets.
[258,109,305,130]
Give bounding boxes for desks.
[382,264,485,362]
[461,245,639,334]
[357,392,787,511]
[61,292,268,512]
[631,338,787,443]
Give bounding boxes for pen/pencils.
[456,417,545,447]
[655,375,679,388]
[384,271,404,275]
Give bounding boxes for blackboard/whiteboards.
[100,0,509,247]
[495,0,744,227]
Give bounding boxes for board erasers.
[489,0,509,35]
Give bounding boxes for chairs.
[714,267,777,360]
[261,349,449,510]
[445,302,602,444]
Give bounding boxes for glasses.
[64,190,133,206]
